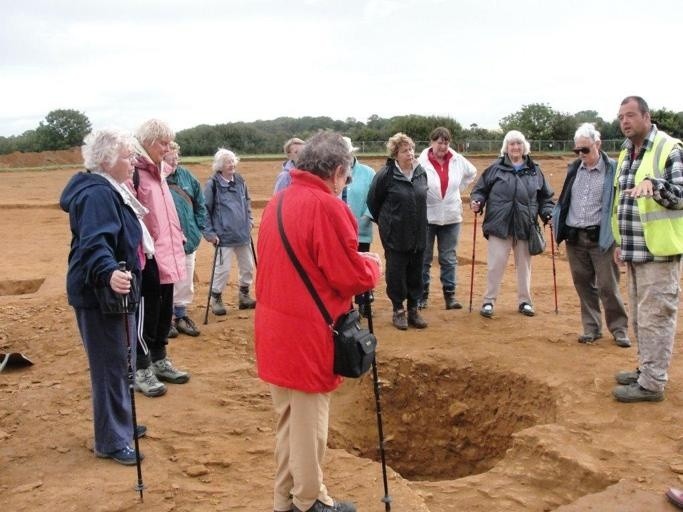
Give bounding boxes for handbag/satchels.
[529,223,546,255]
[335,309,378,378]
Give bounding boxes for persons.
[118,118,192,399]
[608,94,683,404]
[415,125,477,311]
[366,131,432,332]
[254,135,379,512]
[202,146,258,317]
[336,136,376,318]
[163,141,209,340]
[468,129,556,318]
[550,121,633,349]
[270,138,305,195]
[58,123,148,465]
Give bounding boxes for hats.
[342,136,360,153]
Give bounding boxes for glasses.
[118,151,137,162]
[344,169,352,184]
[572,142,596,154]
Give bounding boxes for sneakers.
[94,444,144,465]
[612,330,631,347]
[519,303,535,316]
[151,356,190,384]
[612,370,665,402]
[167,315,200,338]
[579,333,602,343]
[392,298,428,330]
[445,295,463,309]
[133,425,147,439]
[134,366,167,396]
[480,303,493,317]
[665,487,683,509]
[292,499,358,512]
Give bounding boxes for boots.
[239,286,256,309]
[209,291,227,315]
